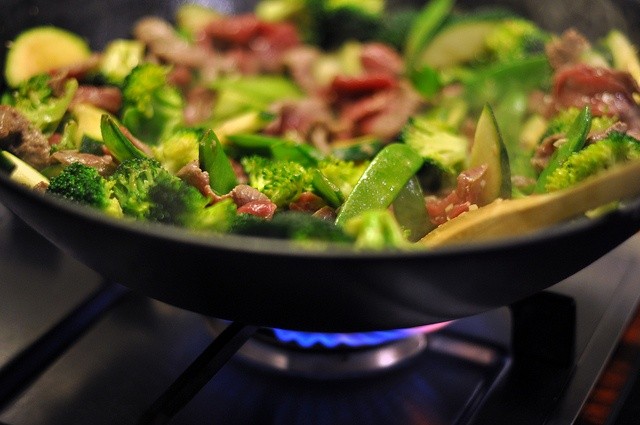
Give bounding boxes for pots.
[2,2,640,331]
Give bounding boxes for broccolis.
[1,3,640,240]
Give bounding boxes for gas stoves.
[0,0,640,425]
